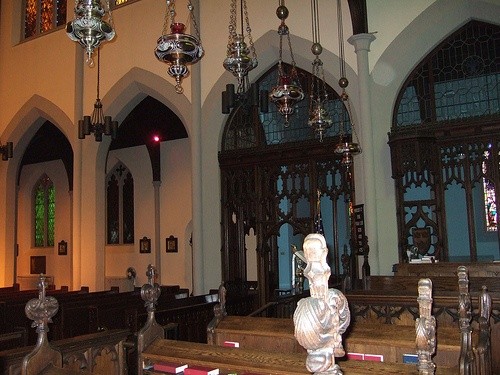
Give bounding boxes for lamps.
[217,0,259,97]
[66,0,116,68]
[154,0,205,94]
[269,0,304,128]
[308,0,333,141]
[336,0,362,174]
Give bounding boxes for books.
[363,354,384,362]
[184,366,220,375]
[224,341,240,348]
[345,353,363,360]
[402,354,418,363]
[154,361,188,373]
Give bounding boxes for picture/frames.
[138,236,152,254]
[164,235,180,253]
[57,240,68,256]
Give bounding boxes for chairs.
[0,263,500,375]
